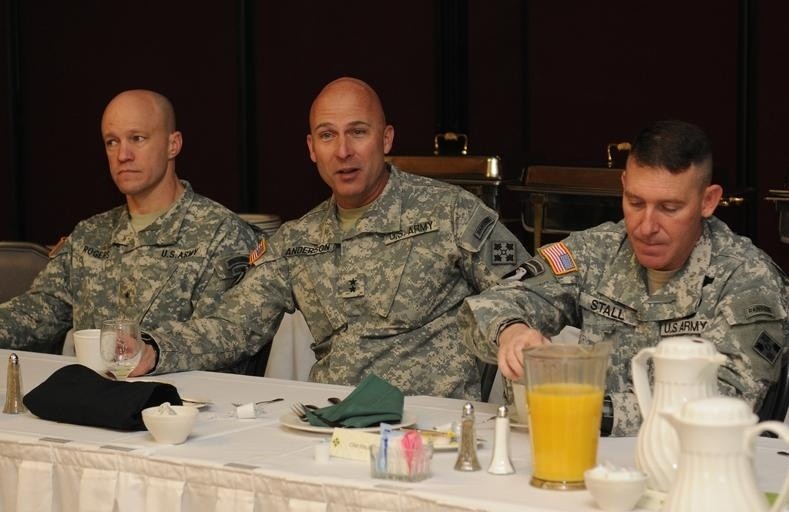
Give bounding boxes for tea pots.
[630,336,729,507]
[657,396,789,512]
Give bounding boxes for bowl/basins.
[141,405,200,446]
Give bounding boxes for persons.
[102,75,540,404]
[0,87,269,377]
[457,116,789,438]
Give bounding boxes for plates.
[279,410,419,434]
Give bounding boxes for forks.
[290,403,347,432]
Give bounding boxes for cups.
[517,341,612,492]
[579,466,648,511]
[72,328,116,375]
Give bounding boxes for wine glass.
[100,319,144,381]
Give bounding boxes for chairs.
[0,242,67,356]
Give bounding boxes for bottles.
[3,351,27,419]
[453,403,515,476]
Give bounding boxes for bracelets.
[600,395,614,437]
[140,332,160,374]
